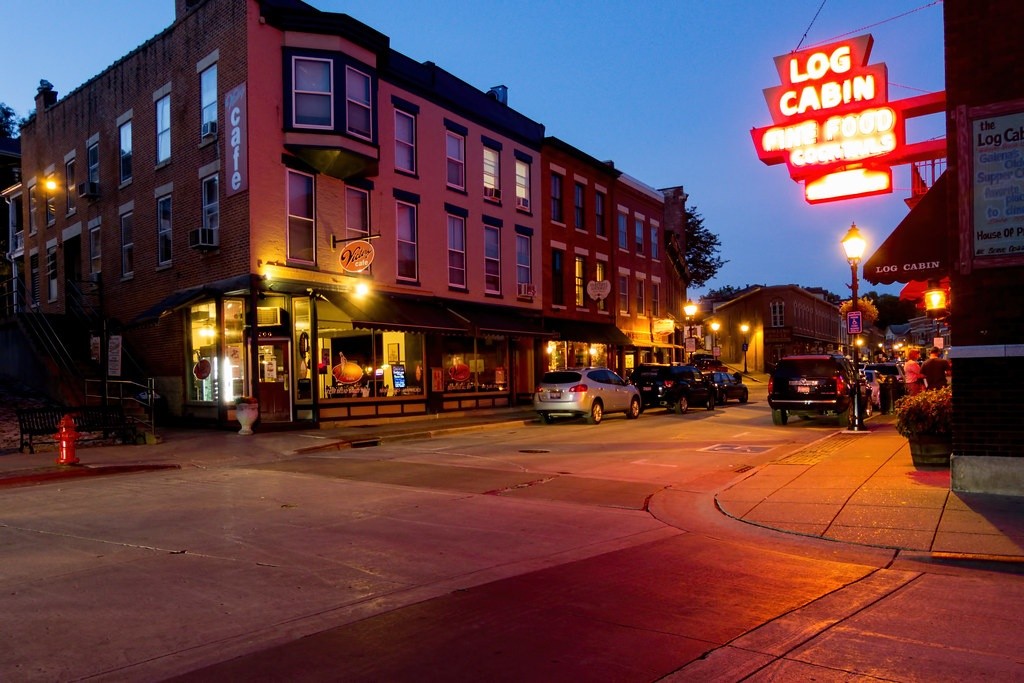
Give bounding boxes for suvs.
[765,352,874,427]
[631,361,717,416]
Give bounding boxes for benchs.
[16,403,138,454]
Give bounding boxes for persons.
[905,350,926,396]
[920,347,951,390]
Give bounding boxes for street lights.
[740,324,750,374]
[684,299,697,367]
[842,220,869,431]
[711,321,720,360]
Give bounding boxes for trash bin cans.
[879,376,906,415]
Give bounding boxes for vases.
[909,433,951,470]
[235,403,258,435]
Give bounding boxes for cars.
[851,360,924,410]
[689,352,728,373]
[702,371,748,406]
[533,367,642,425]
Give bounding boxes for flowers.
[892,385,951,438]
[233,396,257,404]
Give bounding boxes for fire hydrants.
[53,415,81,466]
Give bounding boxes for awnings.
[125,289,205,332]
[316,291,633,344]
[863,168,949,286]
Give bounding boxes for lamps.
[923,277,948,317]
